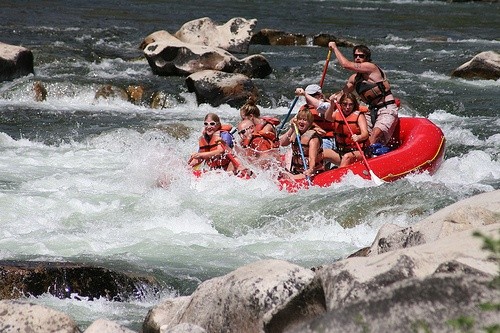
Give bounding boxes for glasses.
[238,126,251,135]
[353,53,365,59]
[204,121,218,126]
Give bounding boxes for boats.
[190,117,445,195]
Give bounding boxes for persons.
[239,102,278,148]
[237,119,270,154]
[188,111,240,176]
[279,108,324,177]
[276,83,334,168]
[328,41,398,160]
[315,93,369,168]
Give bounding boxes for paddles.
[276,91,300,137]
[319,45,333,98]
[333,99,389,187]
[293,121,312,190]
[187,157,193,165]
[220,143,250,179]
[231,127,239,134]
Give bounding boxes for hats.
[304,84,323,96]
